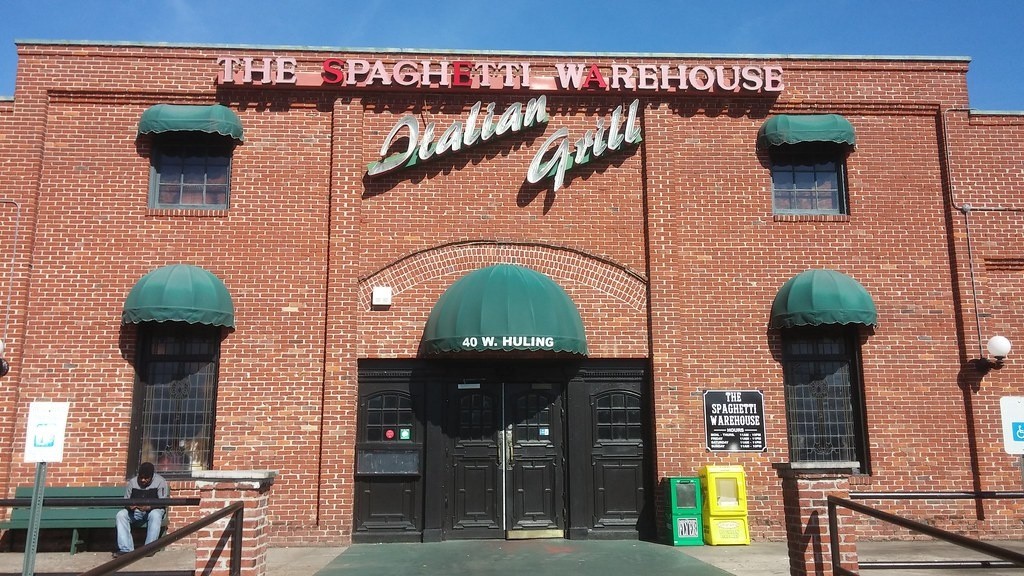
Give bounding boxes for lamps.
[0,339,9,376]
[987,335,1011,368]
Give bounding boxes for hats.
[139,462,154,479]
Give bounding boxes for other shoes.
[145,549,161,557]
[113,551,128,558]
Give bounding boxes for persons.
[113,462,168,557]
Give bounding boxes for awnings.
[123,264,235,328]
[769,269,877,329]
[138,104,244,141]
[757,115,856,147]
[423,265,588,356]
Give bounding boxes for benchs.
[0,485,171,555]
